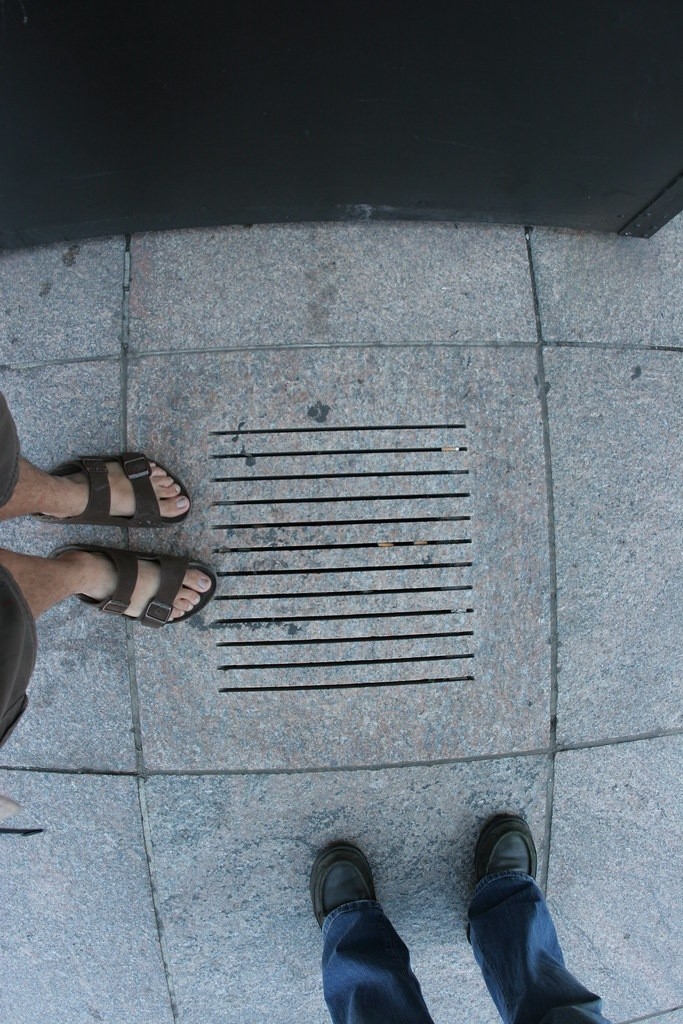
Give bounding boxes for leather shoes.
[309,843,377,930]
[473,815,538,883]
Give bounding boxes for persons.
[309,813,613,1024]
[0,390,216,750]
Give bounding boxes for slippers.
[45,543,219,629]
[29,452,191,526]
